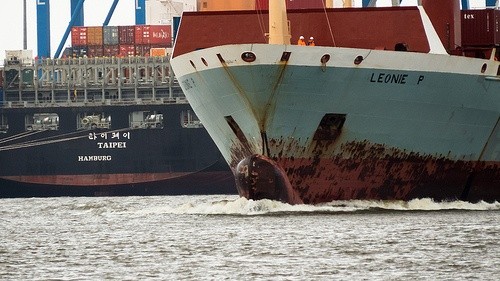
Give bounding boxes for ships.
[167,1,500,205]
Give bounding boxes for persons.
[308,36,316,45]
[296,35,307,46]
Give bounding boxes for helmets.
[309,37,314,39]
[300,36,304,39]
